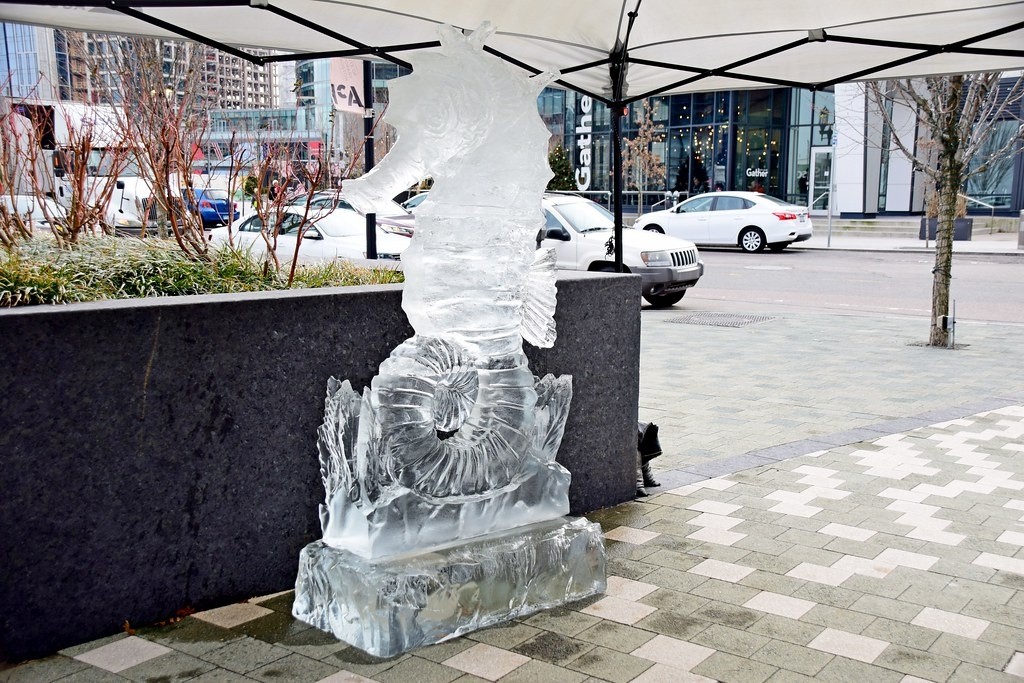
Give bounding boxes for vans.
[1,94,193,237]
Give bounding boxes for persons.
[748,180,764,192]
[693,177,709,194]
[658,181,665,205]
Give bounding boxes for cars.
[271,187,631,260]
[540,193,706,308]
[180,183,240,228]
[2,192,68,229]
[630,189,815,254]
[207,207,412,273]
